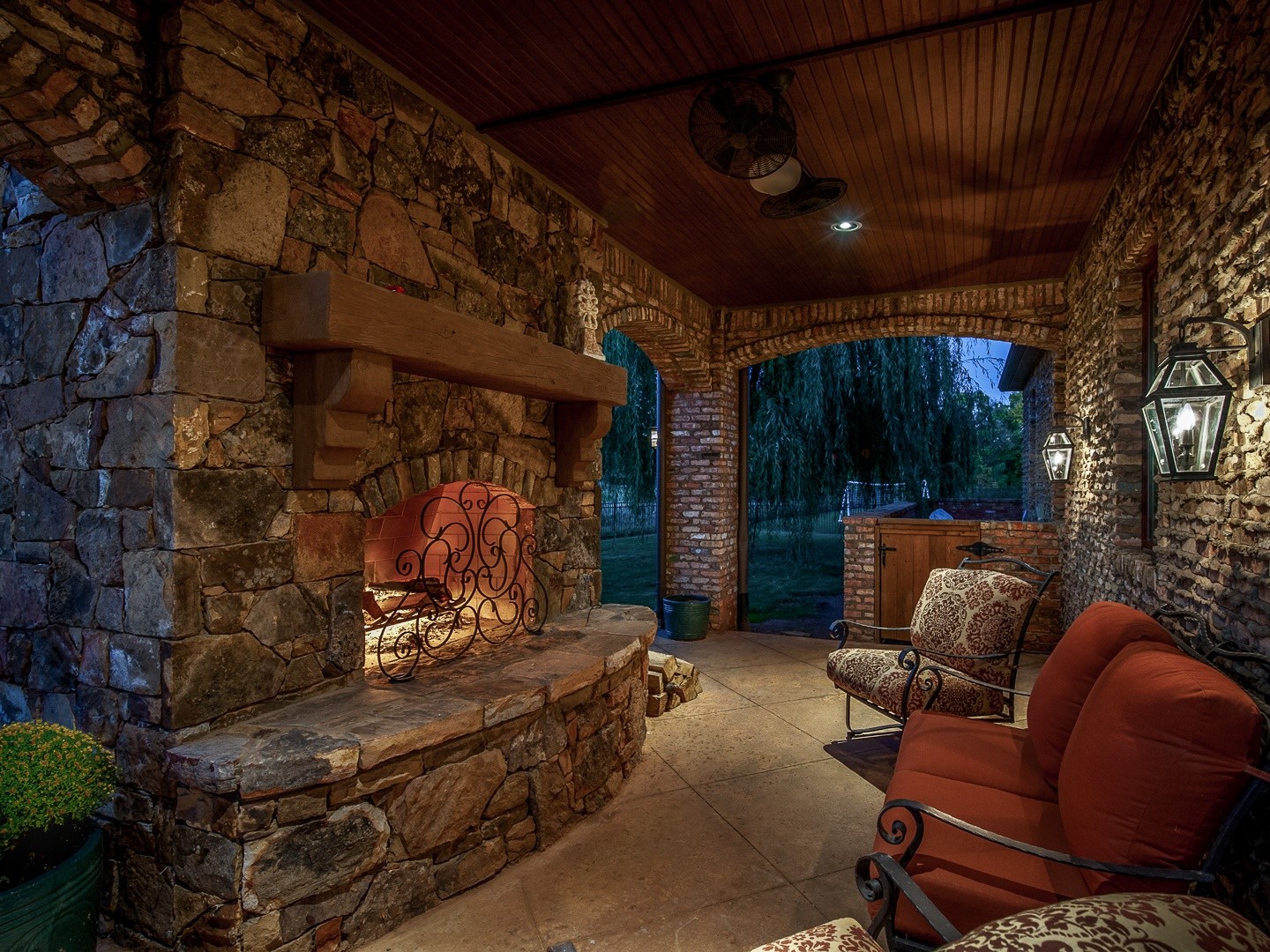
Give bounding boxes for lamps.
[1139,311,1257,481]
[1042,411,1088,482]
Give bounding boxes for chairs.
[827,545,1063,751]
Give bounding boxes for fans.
[687,65,848,221]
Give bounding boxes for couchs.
[856,585,1270,943]
[743,798,1269,951]
